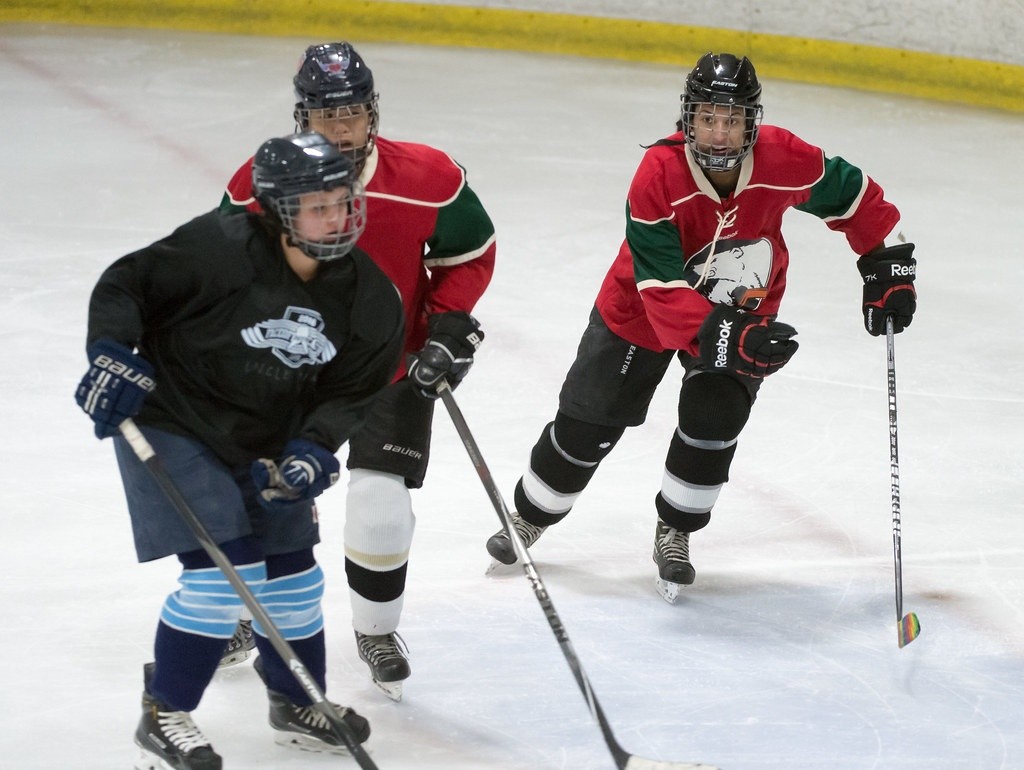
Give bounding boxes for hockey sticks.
[420,335,720,770]
[884,313,923,649]
[118,415,380,770]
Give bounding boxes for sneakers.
[215,620,257,670]
[254,655,371,757]
[487,511,548,573]
[355,630,411,702]
[651,517,695,603]
[134,663,222,770]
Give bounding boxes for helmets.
[679,52,763,172]
[292,42,379,163]
[252,130,368,260]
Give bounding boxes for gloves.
[698,303,798,378]
[855,243,917,336]
[74,339,153,440]
[408,311,485,399]
[252,439,340,510]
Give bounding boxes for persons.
[487,50,915,603]
[220,42,499,703]
[74,130,406,770]
